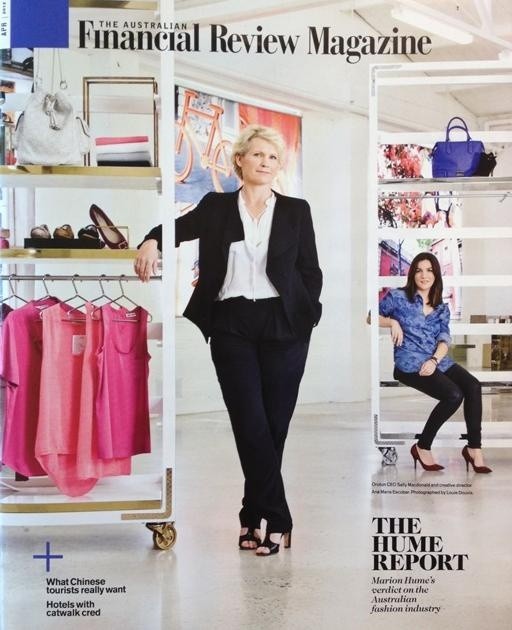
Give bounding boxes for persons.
[362,250,492,477]
[133,121,324,558]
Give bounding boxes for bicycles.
[174,85,241,197]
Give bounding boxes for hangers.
[1,273,155,326]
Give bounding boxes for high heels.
[237,517,263,550]
[461,445,494,474]
[27,203,130,250]
[470,151,499,178]
[254,523,294,556]
[410,443,447,472]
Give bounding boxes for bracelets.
[429,360,437,366]
[431,356,441,364]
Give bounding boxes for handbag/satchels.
[427,114,486,178]
[15,90,94,167]
